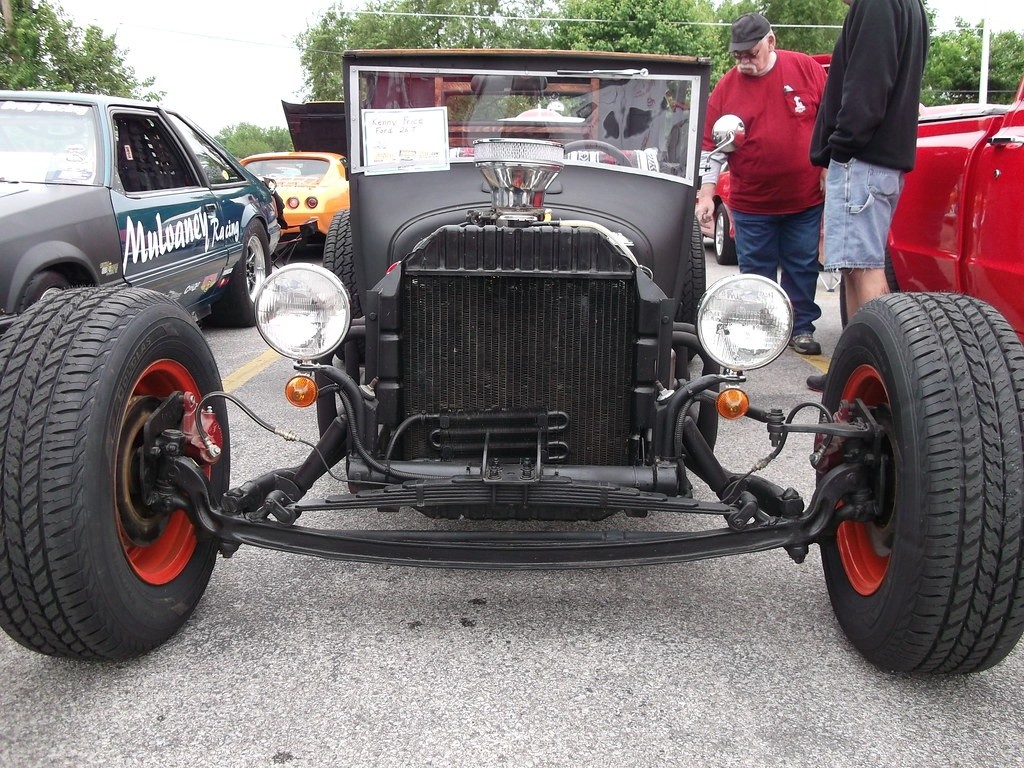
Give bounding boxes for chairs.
[264,178,319,269]
[117,126,143,191]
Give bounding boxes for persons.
[696,13,830,354]
[806,0,930,394]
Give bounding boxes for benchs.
[448,145,660,173]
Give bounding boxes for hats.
[728,12,770,53]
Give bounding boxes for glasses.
[734,34,771,60]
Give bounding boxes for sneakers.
[807,374,828,391]
[789,332,821,355]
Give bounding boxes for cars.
[0,85,283,352]
[689,52,1024,349]
[1,44,1024,677]
[236,148,351,253]
